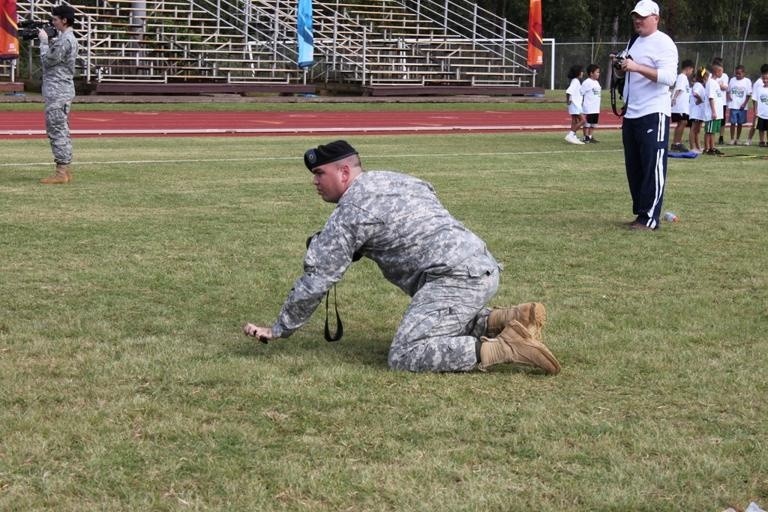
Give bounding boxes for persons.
[688,66,710,154]
[581,64,602,144]
[237,137,567,377]
[611,0,681,231]
[725,65,752,147]
[36,4,82,184]
[700,64,727,157]
[753,69,768,149]
[745,60,768,147]
[566,63,589,146]
[672,60,694,153]
[711,57,730,145]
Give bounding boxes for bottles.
[664,212,678,223]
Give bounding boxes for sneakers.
[566,132,599,145]
[672,141,767,157]
[38,174,73,185]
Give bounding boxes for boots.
[481,321,562,375]
[488,301,545,343]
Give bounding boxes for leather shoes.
[627,218,656,232]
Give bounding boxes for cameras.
[614,51,633,70]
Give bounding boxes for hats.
[628,0,660,17]
[305,140,361,173]
